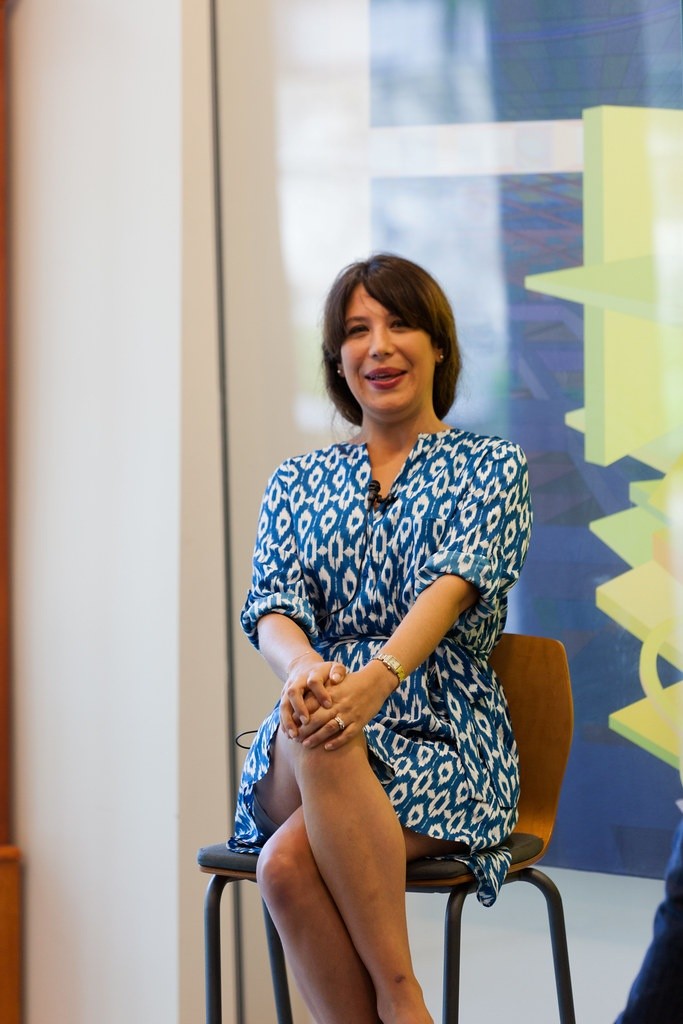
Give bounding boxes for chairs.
[196,630,581,1023]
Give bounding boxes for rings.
[335,717,345,730]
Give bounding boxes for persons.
[609,821,683,1024]
[225,255,534,1024]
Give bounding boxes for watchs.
[371,653,406,687]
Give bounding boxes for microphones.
[366,480,381,512]
